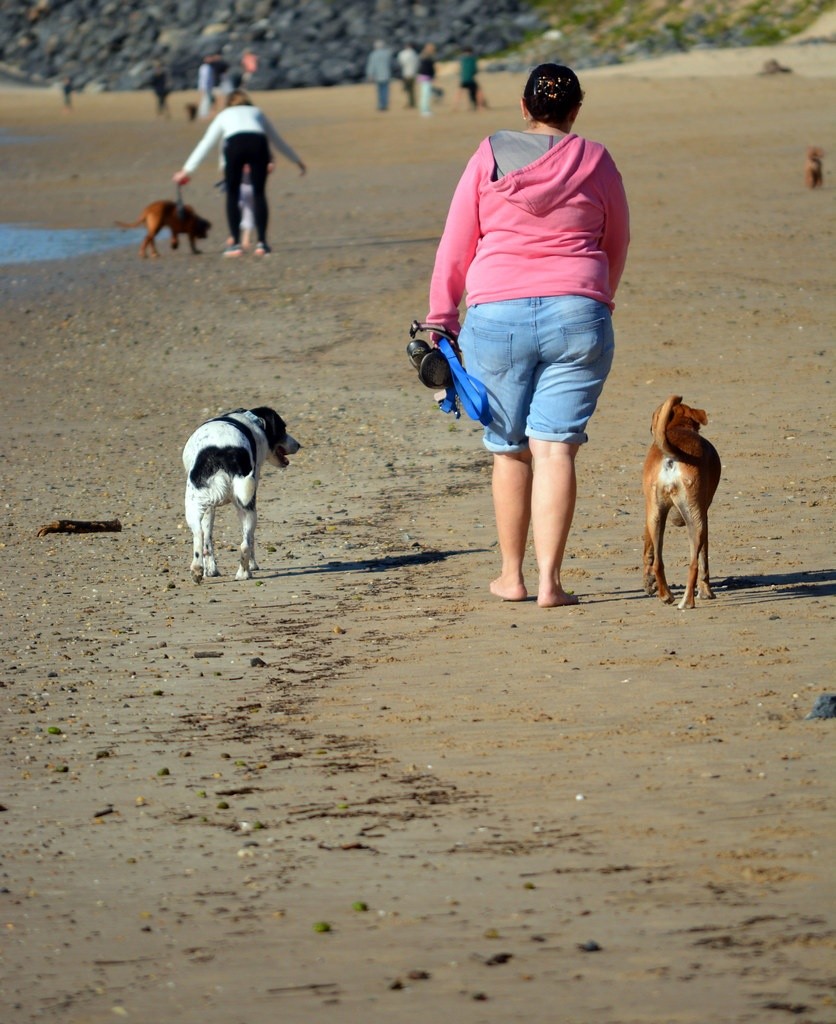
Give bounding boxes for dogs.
[803,147,824,188]
[641,395,723,609]
[430,87,445,100]
[182,407,301,582]
[184,103,198,121]
[112,199,213,259]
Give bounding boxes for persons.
[427,64,630,605]
[172,89,308,257]
[59,43,483,116]
